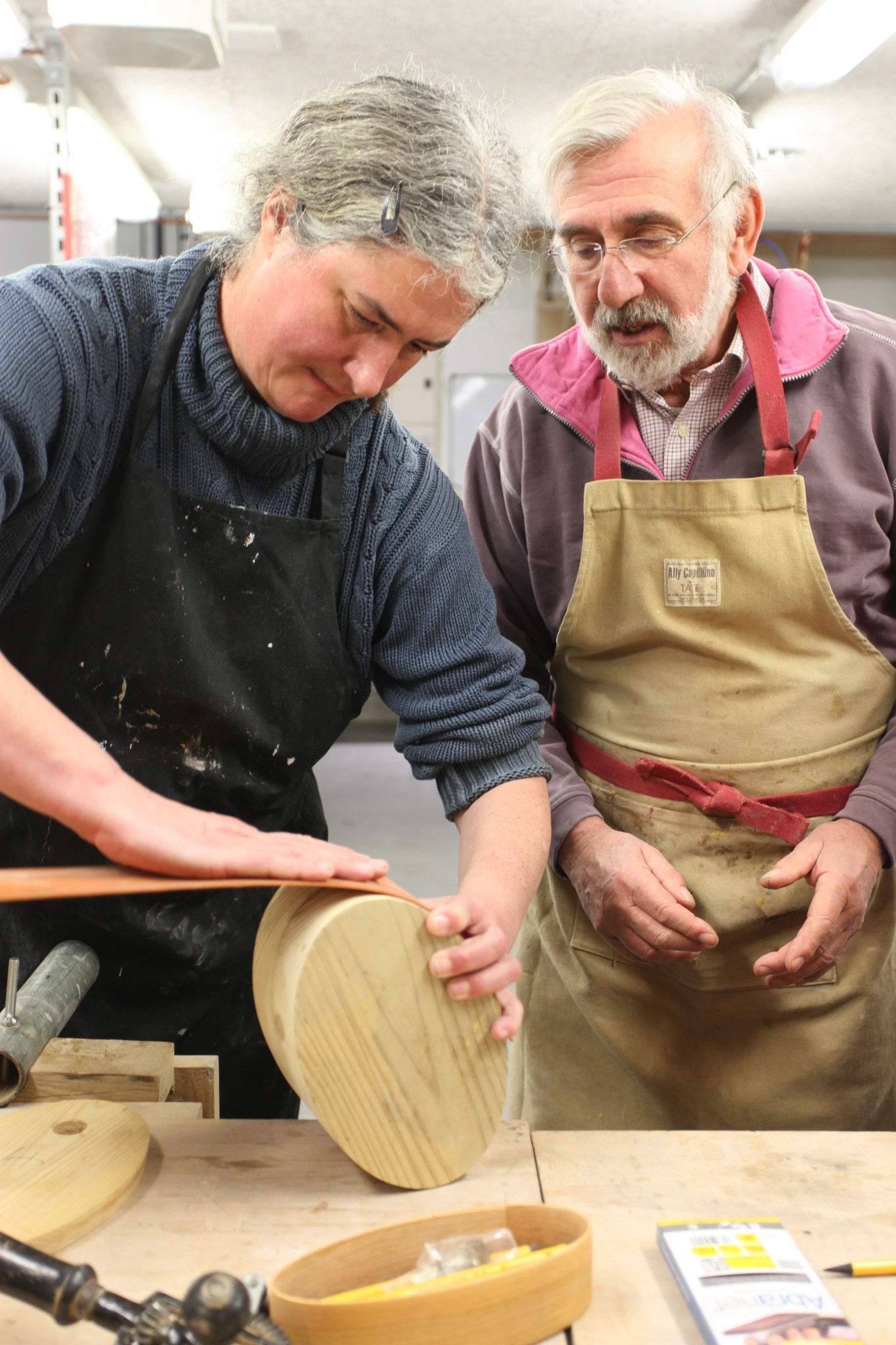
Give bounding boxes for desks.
[533,1129,896,1344]
[1,1118,568,1345]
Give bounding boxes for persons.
[0,77,551,1125]
[457,66,895,1134]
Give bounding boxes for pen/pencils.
[824,1259,896,1276]
[321,1245,536,1301]
[348,1241,574,1303]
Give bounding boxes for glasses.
[552,180,739,276]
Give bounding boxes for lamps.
[761,0,896,89]
